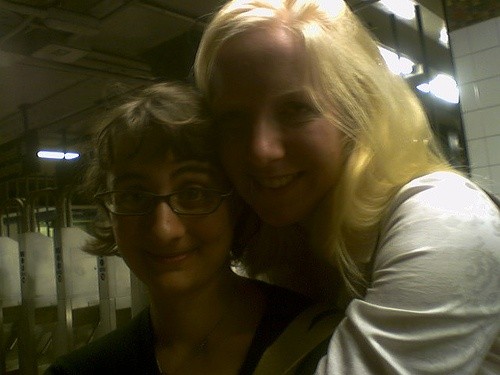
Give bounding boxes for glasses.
[94,187,232,215]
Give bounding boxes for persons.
[43,80,346,375]
[192,0,500,375]
